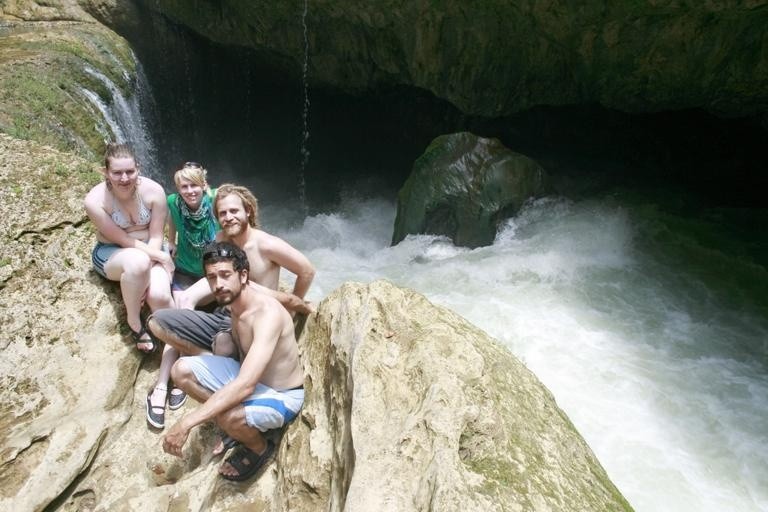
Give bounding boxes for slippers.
[215,429,275,481]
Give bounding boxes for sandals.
[129,325,161,352]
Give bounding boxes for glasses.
[202,248,241,260]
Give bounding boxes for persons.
[160,241,306,482]
[145,161,220,429]
[213,182,314,318]
[83,143,175,354]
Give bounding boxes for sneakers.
[169,386,187,410]
[146,390,165,429]
